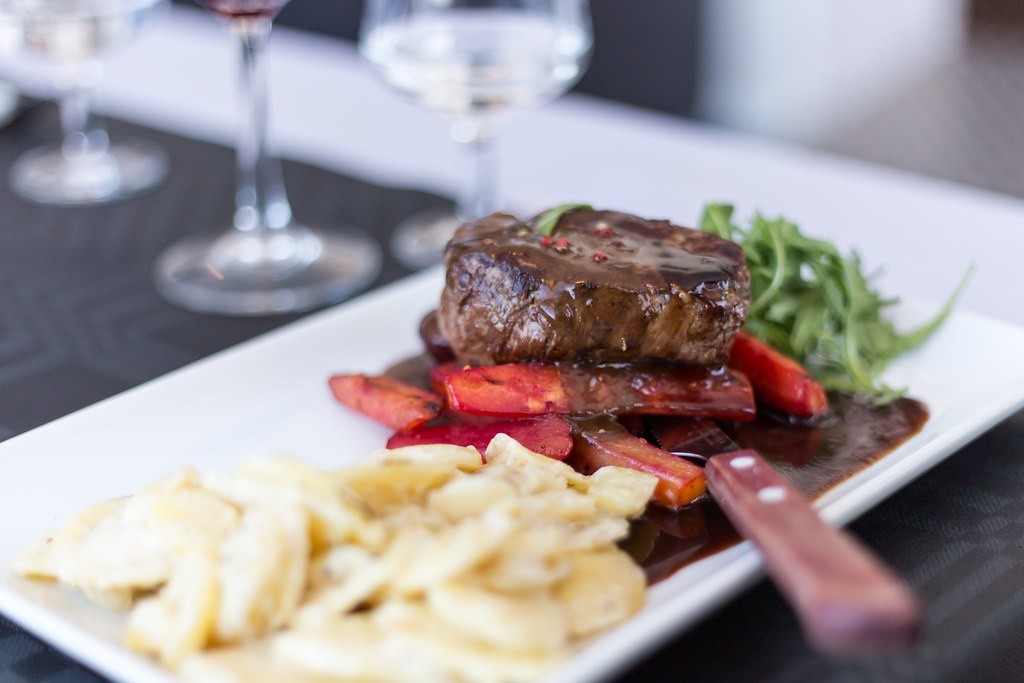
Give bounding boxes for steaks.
[439,201,751,368]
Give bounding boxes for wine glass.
[148,0,381,316]
[356,1,595,270]
[0,0,171,209]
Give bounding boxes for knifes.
[645,415,920,656]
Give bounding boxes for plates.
[0,247,1022,683]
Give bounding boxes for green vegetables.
[536,200,966,406]
